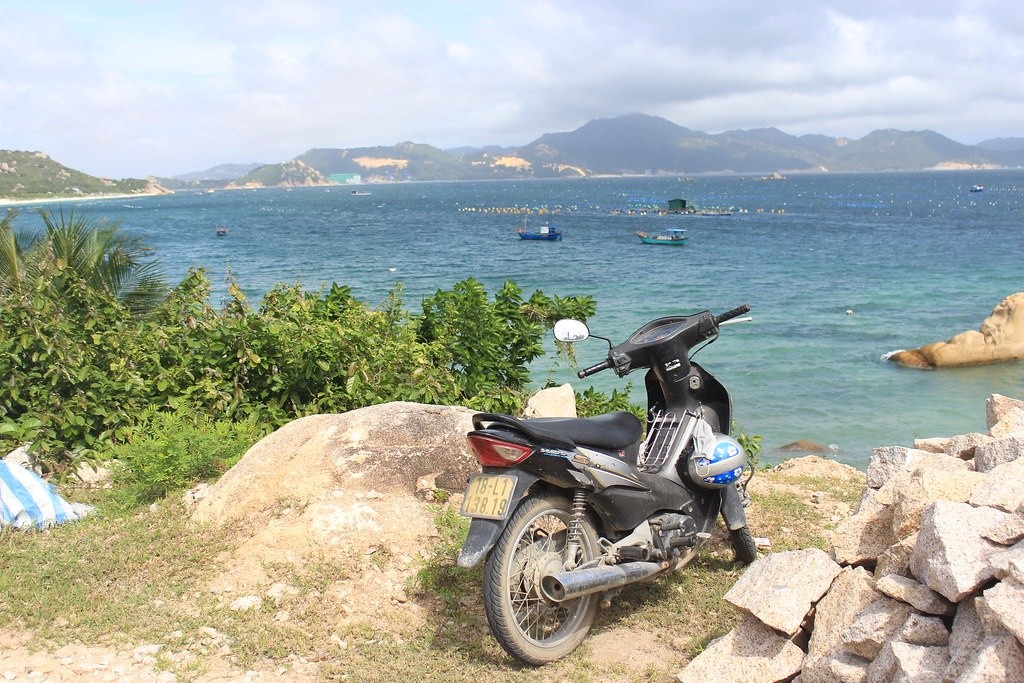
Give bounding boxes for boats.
[145,251,156,254]
[633,228,689,247]
[216,225,228,237]
[970,185,984,193]
[515,221,564,242]
[352,190,370,196]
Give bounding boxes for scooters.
[456,303,756,662]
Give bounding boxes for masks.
[690,418,715,476]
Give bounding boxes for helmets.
[687,433,747,489]
